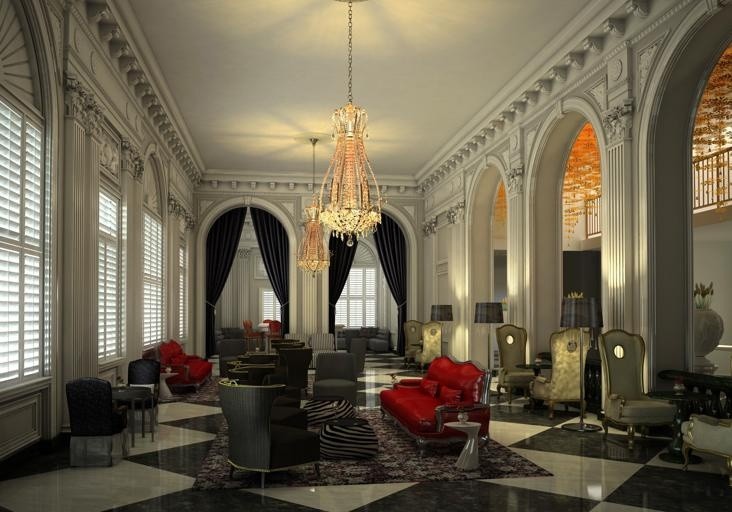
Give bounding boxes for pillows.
[420,378,463,403]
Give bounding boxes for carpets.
[191,405,553,491]
[158,373,316,403]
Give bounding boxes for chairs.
[496,324,535,404]
[679,414,732,470]
[66,376,130,467]
[597,329,677,452]
[216,318,392,489]
[143,349,189,394]
[404,320,442,373]
[529,327,592,420]
[128,357,160,433]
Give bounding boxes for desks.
[515,362,552,409]
[112,389,154,446]
[645,388,715,465]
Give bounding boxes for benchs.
[378,352,494,458]
[155,339,212,395]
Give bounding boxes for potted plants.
[694,280,724,376]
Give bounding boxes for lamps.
[300,139,331,278]
[431,305,453,357]
[316,0,382,247]
[474,302,504,396]
